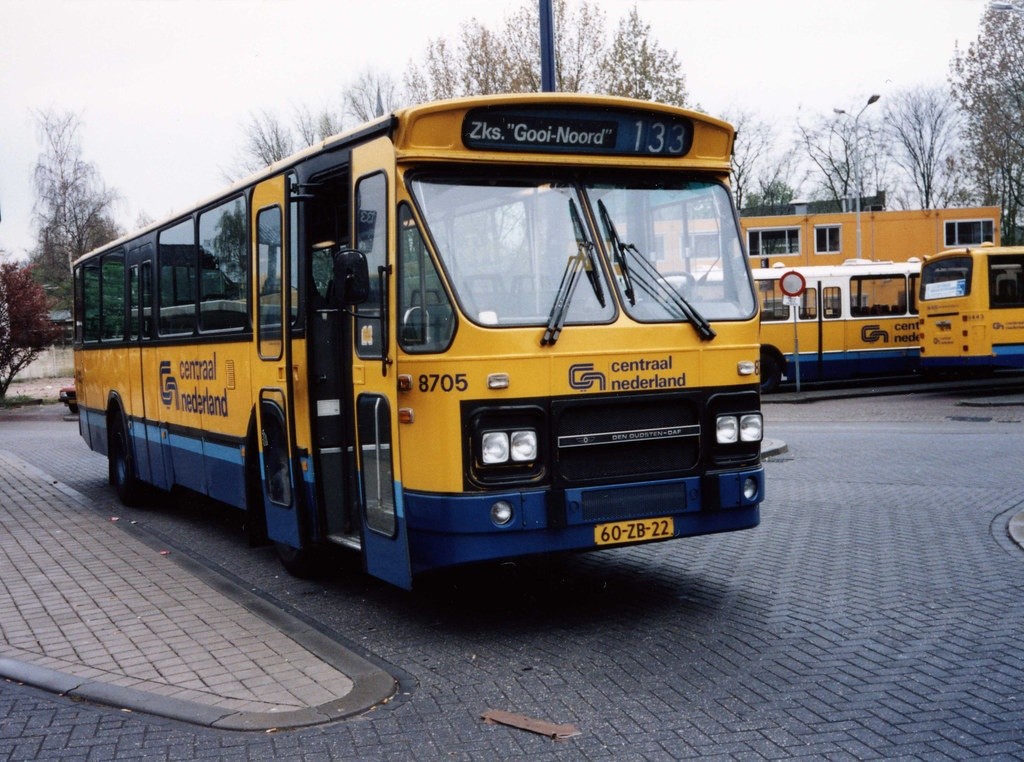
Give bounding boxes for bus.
[918,242,1024,370]
[70,91,766,593]
[658,256,1022,396]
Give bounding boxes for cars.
[58,385,79,415]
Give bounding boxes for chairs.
[409,274,553,347]
[998,280,1016,296]
[762,304,907,321]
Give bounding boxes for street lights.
[834,95,881,259]
